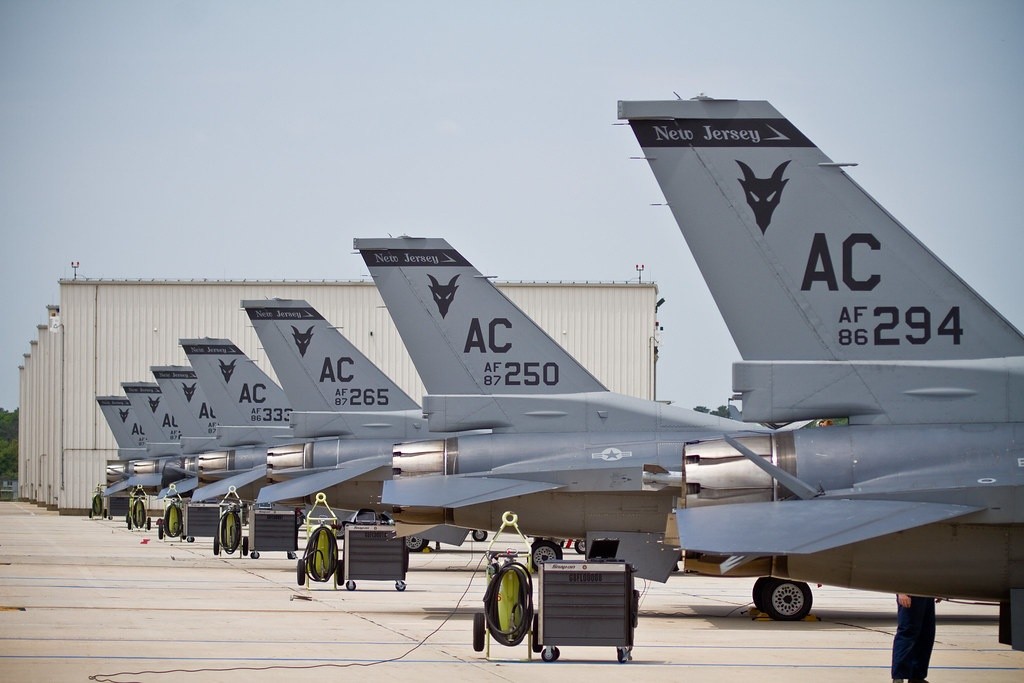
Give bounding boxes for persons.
[891,593,936,683]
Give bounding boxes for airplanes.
[87,86,1023,657]
